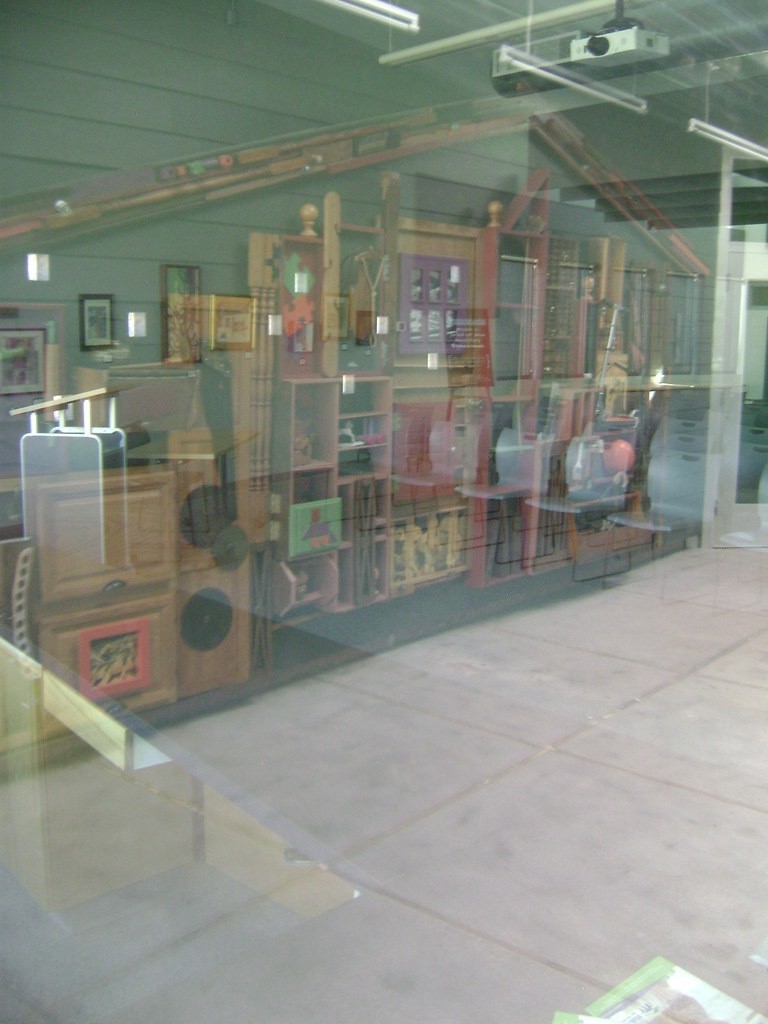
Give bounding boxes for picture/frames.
[78,293,115,349]
[160,264,203,363]
[0,328,47,396]
[209,291,257,353]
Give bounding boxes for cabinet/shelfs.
[320,0,420,36]
[466,228,602,589]
[280,373,394,624]
[0,303,66,424]
[0,461,177,755]
[75,366,196,432]
[170,425,257,701]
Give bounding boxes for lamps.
[499,0,650,115]
[689,61,768,161]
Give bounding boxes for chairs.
[391,398,768,584]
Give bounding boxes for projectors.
[570,26,669,67]
